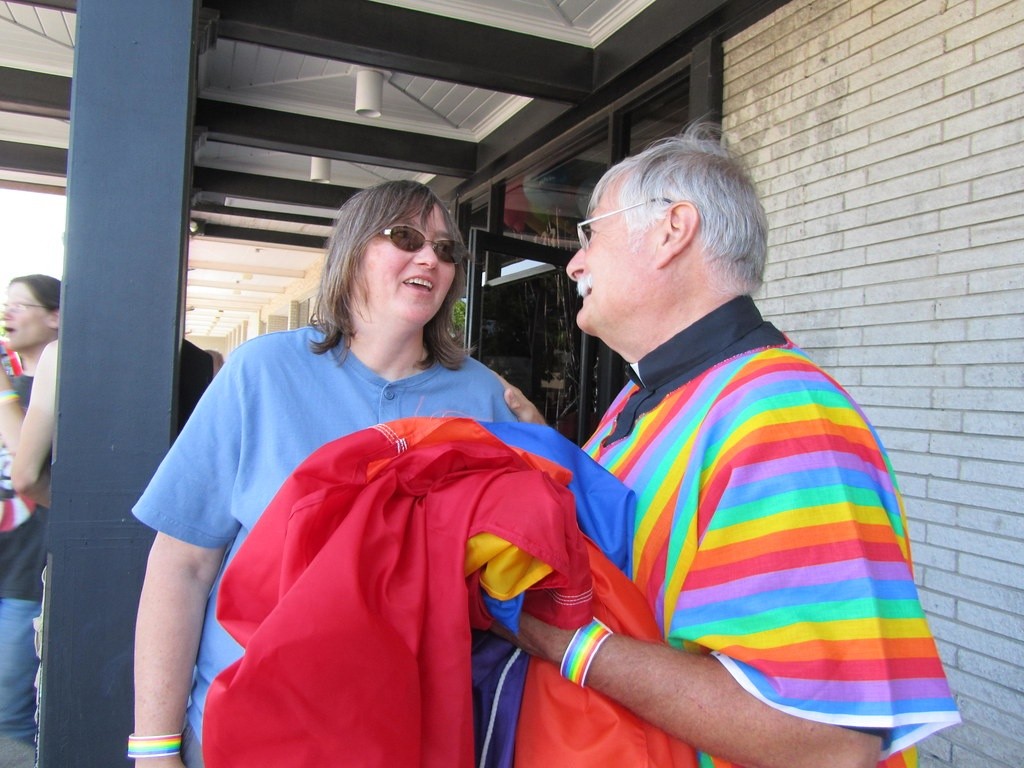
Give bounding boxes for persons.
[480,135,964,767]
[0,273,223,747]
[125,180,524,767]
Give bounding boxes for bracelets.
[560,617,614,688]
[127,733,183,759]
[1,389,21,405]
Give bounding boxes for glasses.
[2,301,46,312]
[380,225,468,264]
[575,196,673,250]
